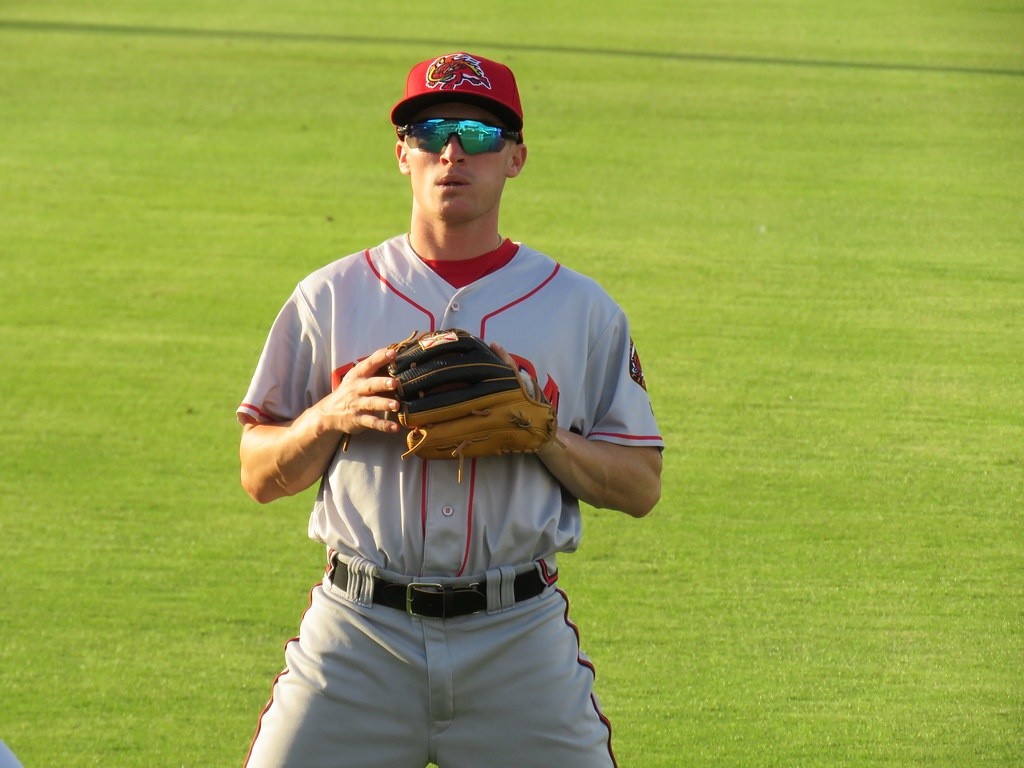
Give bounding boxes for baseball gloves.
[377,327,566,483]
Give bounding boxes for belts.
[330,553,545,620]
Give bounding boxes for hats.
[390,51,524,144]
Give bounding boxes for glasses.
[396,117,520,154]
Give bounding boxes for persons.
[235,51,664,768]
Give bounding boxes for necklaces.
[493,233,502,250]
